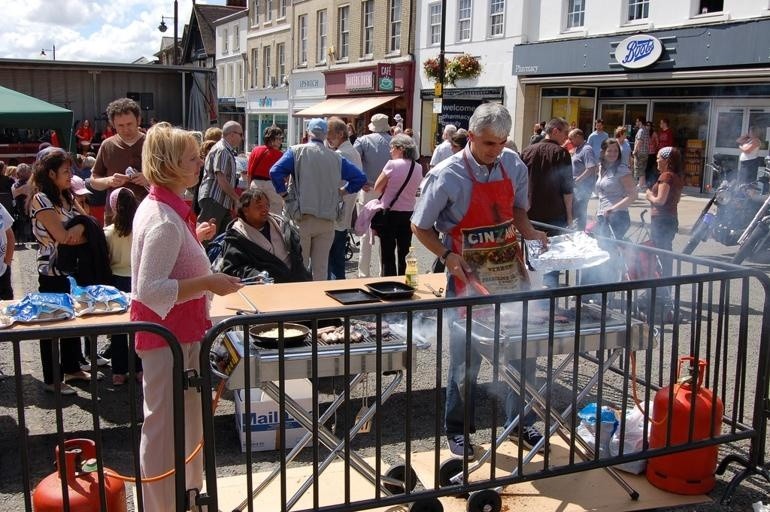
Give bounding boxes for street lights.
[41,45,60,62]
[150,0,182,64]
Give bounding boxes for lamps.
[159,0,182,64]
[40,44,55,60]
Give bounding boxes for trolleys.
[216,315,443,511]
[457,299,658,512]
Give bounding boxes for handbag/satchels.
[371,210,395,240]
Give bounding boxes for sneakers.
[507,425,552,452]
[446,434,475,461]
[112,374,129,386]
[136,371,143,383]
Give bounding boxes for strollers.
[604,209,685,325]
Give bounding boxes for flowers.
[445,54,481,83]
[424,58,448,79]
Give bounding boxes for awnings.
[292,96,397,118]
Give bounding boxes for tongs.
[240,270,274,286]
[227,286,260,315]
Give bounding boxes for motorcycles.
[682,156,768,256]
[724,184,770,263]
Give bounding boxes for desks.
[0,282,256,433]
[241,273,460,405]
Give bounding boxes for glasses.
[275,135,284,140]
[232,131,242,138]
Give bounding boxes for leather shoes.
[65,371,91,383]
[42,382,77,395]
[78,360,92,371]
[85,354,110,365]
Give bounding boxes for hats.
[308,118,328,134]
[368,113,393,133]
[71,176,92,195]
[394,114,402,120]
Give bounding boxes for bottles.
[404,246,420,290]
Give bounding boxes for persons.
[129,120,245,512]
[508,116,685,302]
[186,113,468,282]
[0,96,151,396]
[409,102,552,462]
[733,123,763,185]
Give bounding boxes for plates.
[249,321,311,348]
